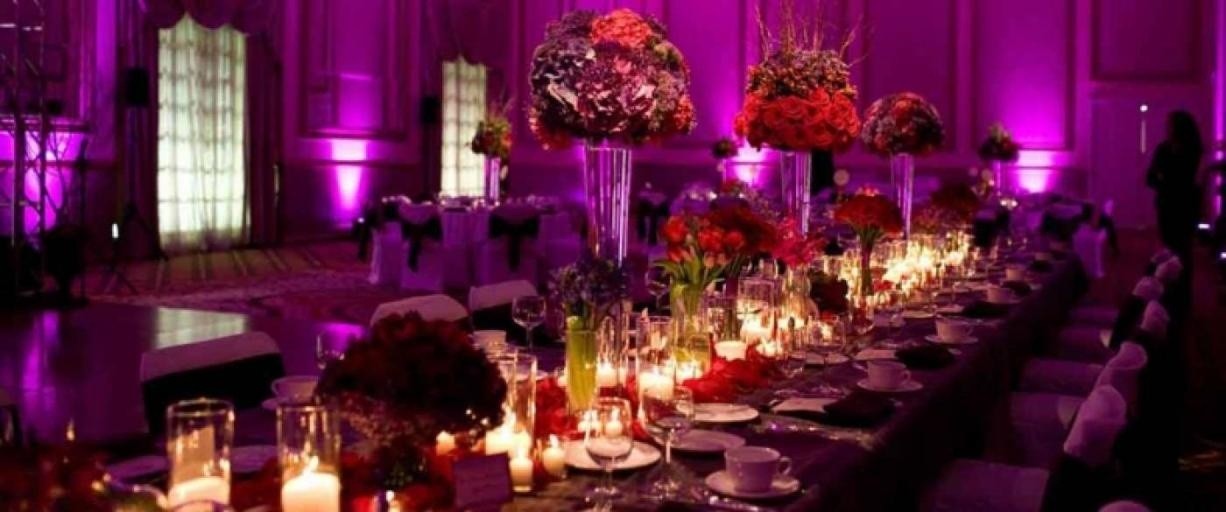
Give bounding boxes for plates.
[563,438,661,469]
[653,427,745,451]
[858,377,922,393]
[674,403,757,422]
[805,351,848,365]
[925,335,978,344]
[263,398,285,409]
[504,364,548,382]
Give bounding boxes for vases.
[783,266,808,323]
[854,248,874,297]
[668,288,711,382]
[780,153,810,235]
[582,145,629,267]
[890,153,913,238]
[987,160,1001,196]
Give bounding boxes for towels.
[654,495,757,512]
[895,343,958,373]
[960,298,1020,321]
[1000,280,1033,297]
[823,386,897,432]
[1027,258,1054,274]
[1048,247,1067,262]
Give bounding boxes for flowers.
[653,201,777,290]
[736,47,860,153]
[860,90,947,153]
[315,312,508,485]
[981,123,1018,160]
[0,436,173,512]
[838,193,903,248]
[778,218,822,267]
[531,9,695,147]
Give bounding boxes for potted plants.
[552,261,629,409]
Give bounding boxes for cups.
[468,330,506,348]
[1034,252,1050,261]
[706,467,801,499]
[812,319,845,395]
[959,253,975,289]
[643,388,694,476]
[586,399,633,502]
[980,250,997,286]
[988,287,1011,304]
[513,296,547,362]
[501,356,536,435]
[1005,269,1026,282]
[921,268,942,314]
[645,267,671,325]
[849,295,875,354]
[709,308,732,344]
[596,316,629,388]
[945,265,961,308]
[888,290,908,329]
[316,332,346,369]
[636,316,678,401]
[760,261,777,281]
[744,280,774,343]
[277,407,341,509]
[724,449,792,493]
[272,374,317,402]
[867,360,910,387]
[167,399,233,512]
[937,321,974,340]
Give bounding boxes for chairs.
[922,382,1126,512]
[991,341,1147,462]
[142,332,288,446]
[483,204,543,282]
[400,204,473,291]
[372,295,472,336]
[1047,275,1165,359]
[470,280,540,340]
[1018,298,1170,393]
[1074,256,1182,324]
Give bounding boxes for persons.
[1145,109,1201,297]
